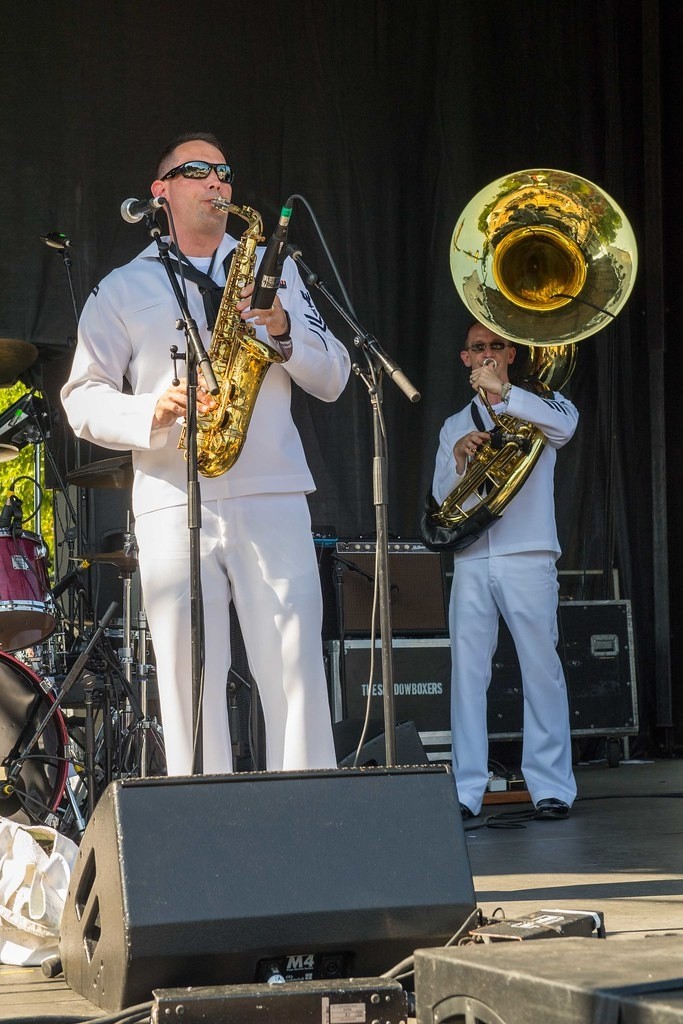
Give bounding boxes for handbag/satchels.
[0,817,80,967]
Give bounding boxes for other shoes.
[535,798,570,818]
[460,802,482,820]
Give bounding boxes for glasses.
[159,161,237,184]
[466,341,510,352]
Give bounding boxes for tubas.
[417,167,639,552]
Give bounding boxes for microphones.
[50,557,95,600]
[0,483,15,530]
[0,780,15,798]
[121,197,166,223]
[40,232,74,248]
[250,198,294,310]
[8,388,37,427]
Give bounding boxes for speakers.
[77,487,124,622]
[332,718,429,768]
[331,541,450,640]
[55,762,479,1014]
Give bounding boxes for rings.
[471,378,475,382]
[271,305,275,314]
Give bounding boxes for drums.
[0,525,70,832]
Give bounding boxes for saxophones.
[174,199,285,478]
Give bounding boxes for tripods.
[112,606,169,778]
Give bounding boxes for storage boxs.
[253,566,641,768]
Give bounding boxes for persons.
[432,322,579,819]
[61,132,351,776]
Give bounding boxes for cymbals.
[0,337,39,383]
[67,551,133,565]
[65,455,133,491]
